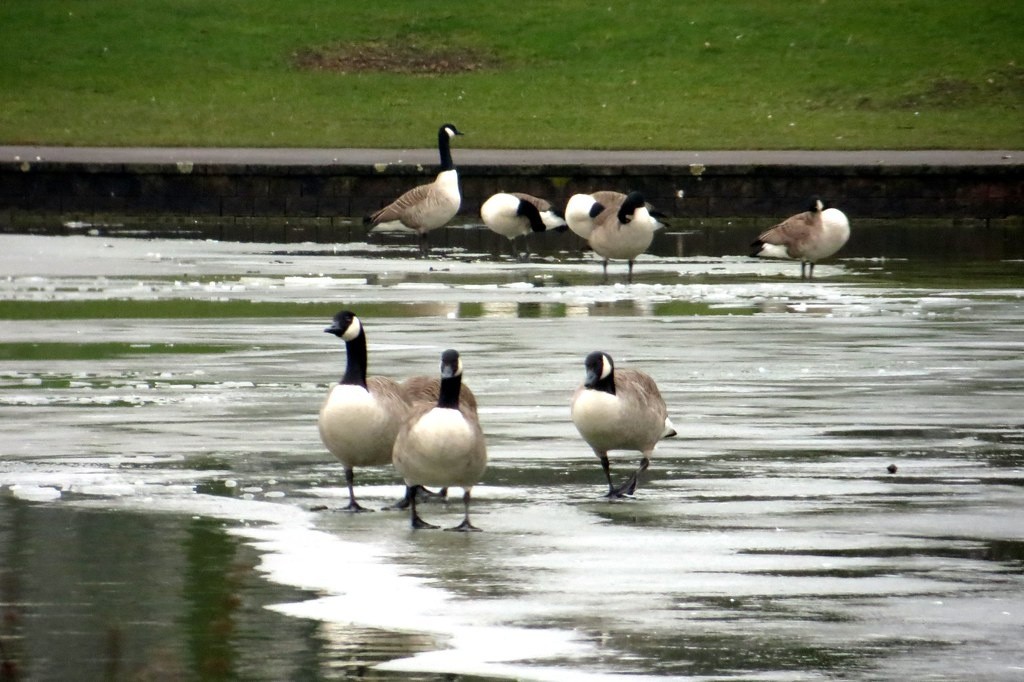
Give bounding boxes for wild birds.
[314,311,413,513]
[364,123,466,250]
[480,193,566,254]
[564,191,664,274]
[573,350,679,499]
[391,349,488,533]
[748,199,851,278]
